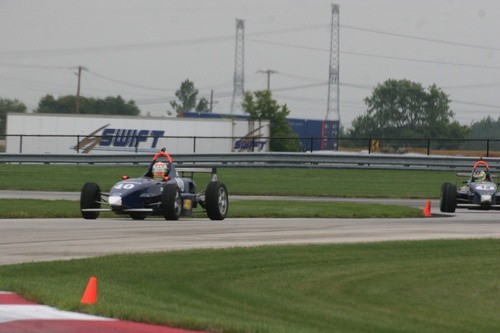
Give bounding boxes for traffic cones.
[423,200,432,216]
[78,276,98,304]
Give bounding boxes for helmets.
[151,162,169,181]
[473,168,486,182]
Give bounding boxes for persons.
[472,168,487,182]
[151,162,169,180]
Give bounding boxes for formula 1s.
[440,158,500,212]
[81,148,228,220]
